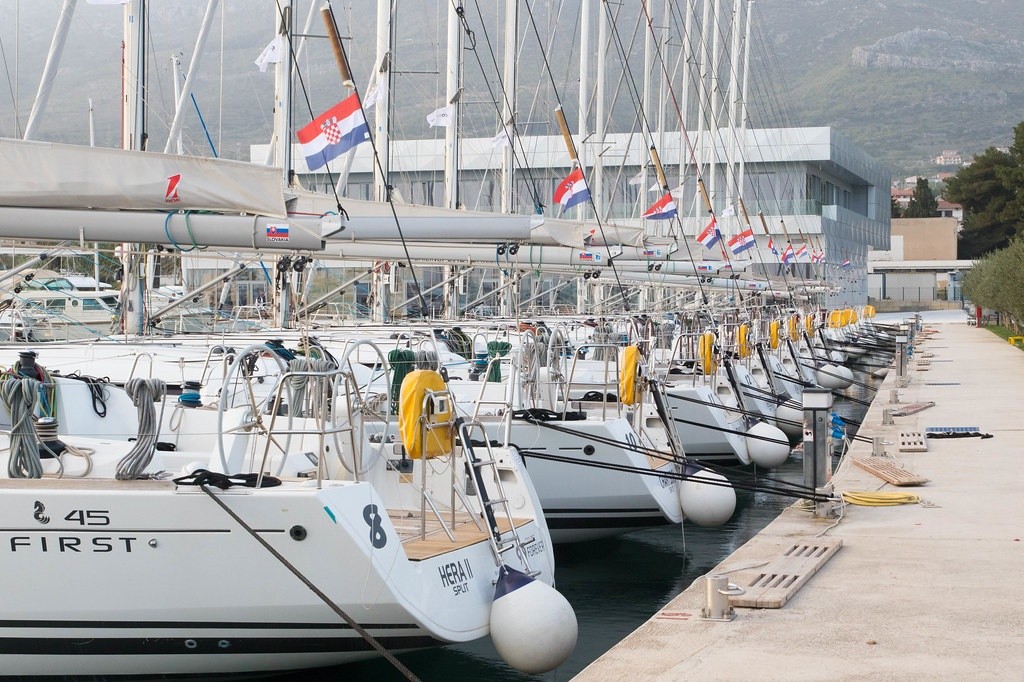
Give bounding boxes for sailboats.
[1,0,919,676]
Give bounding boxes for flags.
[553,169,591,213]
[728,229,755,255]
[297,93,371,170]
[696,218,721,249]
[491,129,507,147]
[812,253,824,262]
[722,207,734,217]
[795,245,807,259]
[768,239,779,255]
[643,193,678,220]
[257,34,283,72]
[781,245,794,261]
[842,259,850,266]
[629,172,641,185]
[426,108,447,127]
